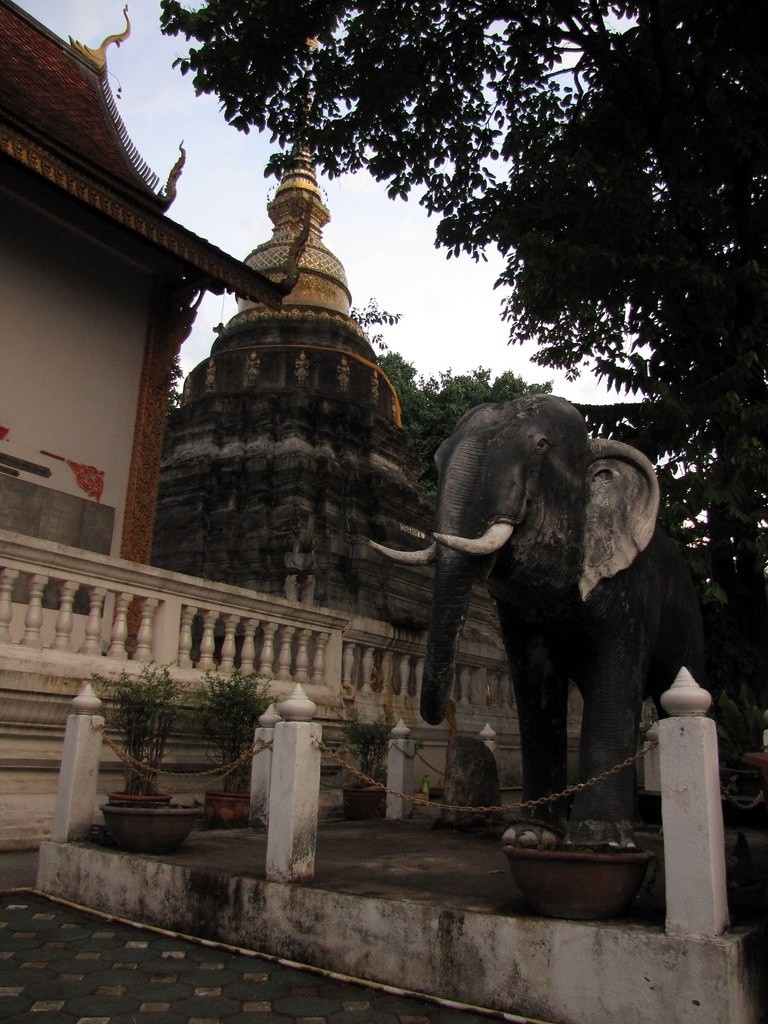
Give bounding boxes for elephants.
[368,393,704,852]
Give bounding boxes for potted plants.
[200,665,288,829]
[90,659,201,808]
[330,687,425,822]
[502,804,652,919]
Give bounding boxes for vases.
[99,804,204,853]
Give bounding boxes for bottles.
[422,775,430,802]
